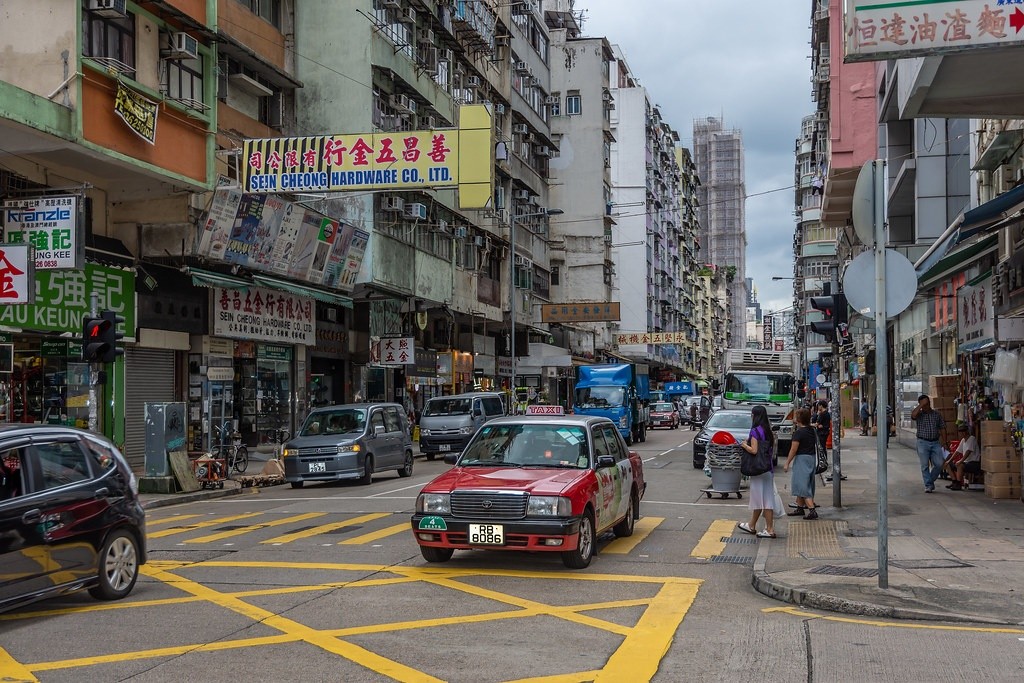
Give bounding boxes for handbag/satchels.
[740,427,772,476]
[815,448,828,474]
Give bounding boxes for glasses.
[958,429,967,432]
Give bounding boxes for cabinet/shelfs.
[241,358,290,446]
[42,359,89,431]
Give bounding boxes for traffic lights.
[810,294,838,338]
[81,309,125,364]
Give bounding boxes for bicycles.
[211,421,249,477]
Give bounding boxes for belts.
[919,437,937,442]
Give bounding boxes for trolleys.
[238,429,290,488]
[192,456,227,490]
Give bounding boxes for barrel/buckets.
[708,443,743,492]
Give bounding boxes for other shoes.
[931,482,935,490]
[951,483,962,490]
[804,510,819,519]
[946,483,956,488]
[787,506,806,516]
[924,485,932,492]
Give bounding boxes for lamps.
[136,264,159,292]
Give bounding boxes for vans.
[284,403,415,488]
[419,392,504,461]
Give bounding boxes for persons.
[911,395,947,493]
[690,403,698,430]
[873,404,893,448]
[784,408,818,520]
[738,406,775,537]
[860,398,871,436]
[810,406,818,429]
[803,404,812,409]
[944,423,980,491]
[818,400,830,459]
[789,408,821,508]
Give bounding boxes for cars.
[694,410,780,469]
[649,400,680,431]
[677,395,722,426]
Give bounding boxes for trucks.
[722,348,806,440]
[570,362,651,446]
[664,381,696,404]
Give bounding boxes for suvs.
[1,423,146,615]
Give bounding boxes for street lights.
[773,277,809,412]
[510,209,564,414]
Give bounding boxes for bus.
[650,390,668,403]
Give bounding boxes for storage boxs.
[929,371,960,448]
[979,418,1021,499]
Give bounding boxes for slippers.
[738,522,756,534]
[757,529,776,538]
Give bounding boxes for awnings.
[914,185,1024,296]
[251,273,353,310]
[605,351,633,362]
[180,267,257,290]
[682,379,708,387]
[970,129,1024,170]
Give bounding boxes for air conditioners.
[388,93,417,115]
[517,256,526,265]
[527,261,533,269]
[403,202,427,220]
[380,196,406,211]
[455,226,467,237]
[421,115,436,127]
[495,186,505,208]
[515,188,547,216]
[436,219,449,231]
[162,29,200,60]
[471,236,483,246]
[417,29,435,43]
[499,209,511,226]
[383,0,402,9]
[992,164,1014,197]
[439,26,616,155]
[88,0,127,19]
[397,7,417,23]
[425,47,438,74]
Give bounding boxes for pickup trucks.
[410,405,646,570]
